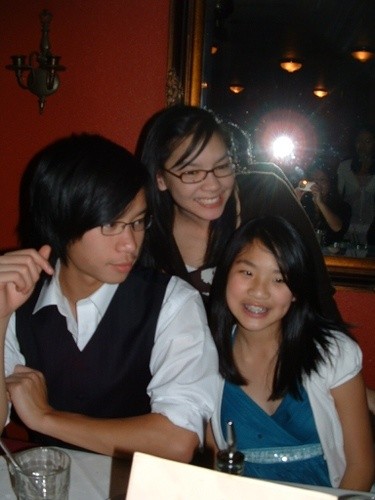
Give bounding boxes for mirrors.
[168,0,375,290]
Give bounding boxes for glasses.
[310,176,329,184]
[165,160,241,184]
[98,212,153,236]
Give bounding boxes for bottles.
[212,419,246,477]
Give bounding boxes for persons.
[230,120,375,261]
[205,214,375,495]
[0,131,219,464]
[134,106,358,341]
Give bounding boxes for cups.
[316,229,368,258]
[6,447,71,499]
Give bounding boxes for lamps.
[279,57,304,74]
[313,88,328,99]
[228,82,245,94]
[351,46,375,64]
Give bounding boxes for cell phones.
[299,180,315,191]
